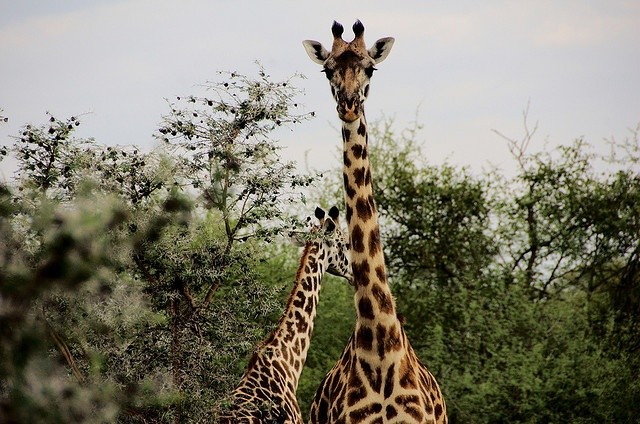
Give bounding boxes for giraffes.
[215,206,355,424]
[302,19,449,424]
